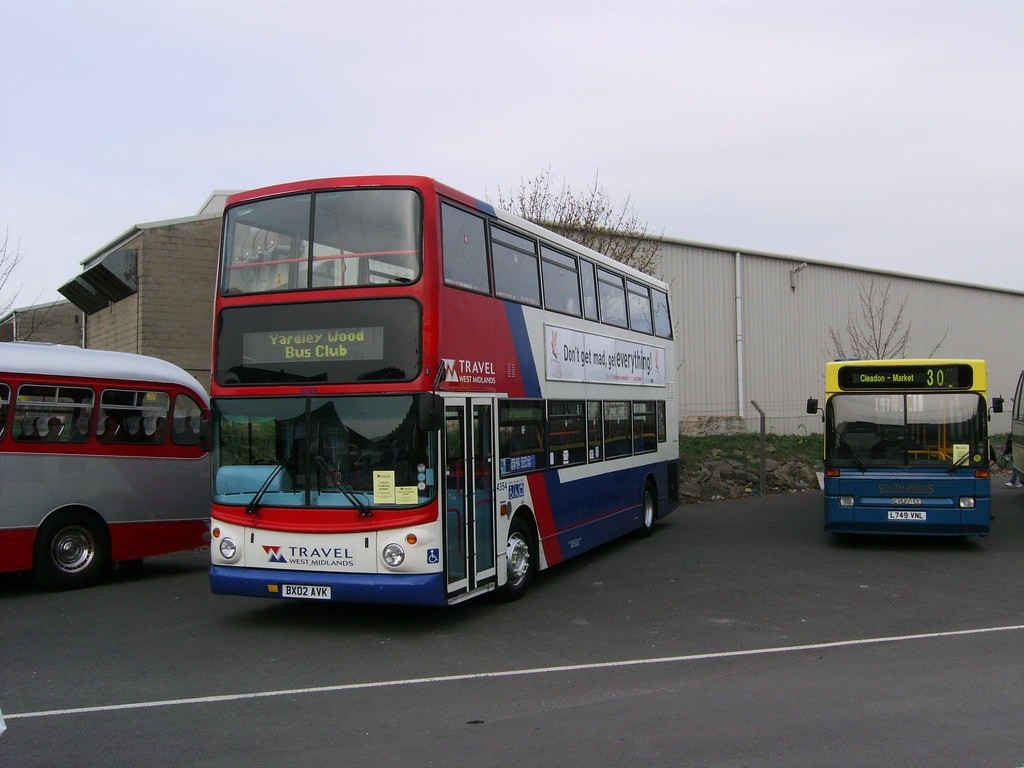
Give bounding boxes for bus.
[1011,370,1024,488]
[1,339,213,594]
[199,176,682,606]
[807,359,1005,541]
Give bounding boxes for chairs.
[447,418,655,490]
[0,408,199,444]
